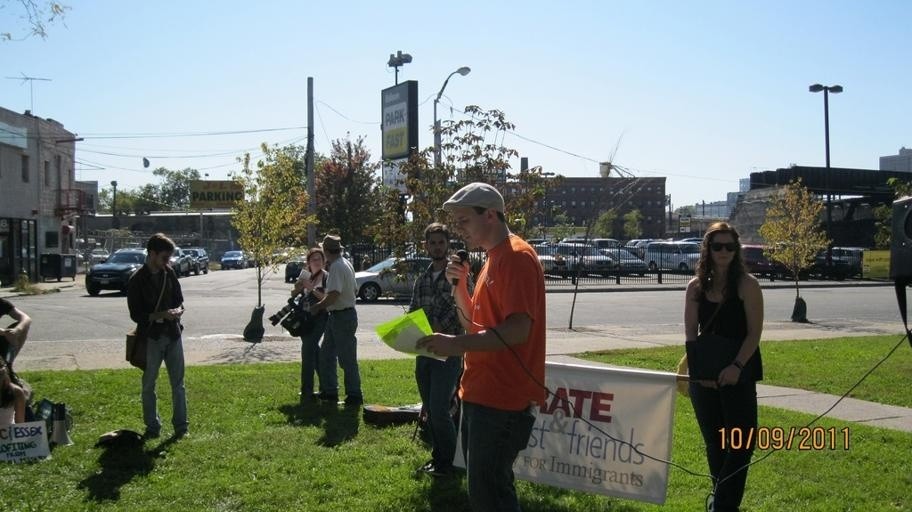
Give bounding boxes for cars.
[90,248,109,263]
[352,239,427,256]
[350,256,433,303]
[526,237,701,281]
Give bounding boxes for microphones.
[446,248,469,304]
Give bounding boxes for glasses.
[711,242,736,252]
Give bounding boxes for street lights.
[111,181,117,225]
[540,172,555,236]
[432,65,472,184]
[808,83,845,281]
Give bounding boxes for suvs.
[86,248,148,295]
[169,247,313,283]
[741,244,872,274]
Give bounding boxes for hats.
[442,182,505,213]
[318,235,344,250]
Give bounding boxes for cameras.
[268,298,298,326]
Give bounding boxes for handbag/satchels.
[281,309,312,337]
[676,355,690,397]
[125,330,146,369]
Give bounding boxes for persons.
[361,254,371,270]
[291,248,330,404]
[416,182,546,511]
[308,234,364,406]
[684,219,763,511]
[408,223,474,479]
[0,355,26,425]
[127,230,190,438]
[0,298,36,421]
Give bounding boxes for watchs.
[310,288,316,294]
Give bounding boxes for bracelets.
[732,358,744,372]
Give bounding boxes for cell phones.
[170,306,184,314]
